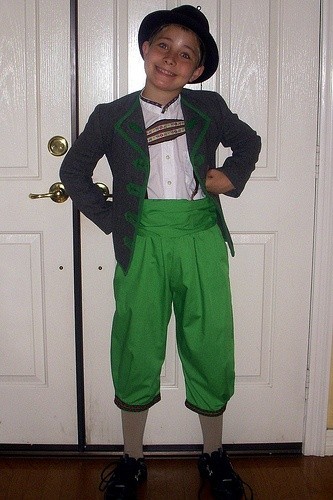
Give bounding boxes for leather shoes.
[198,447,253,500]
[98,454,147,500]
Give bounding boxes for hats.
[139,5,218,84]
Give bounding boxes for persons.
[59,4,262,500]
